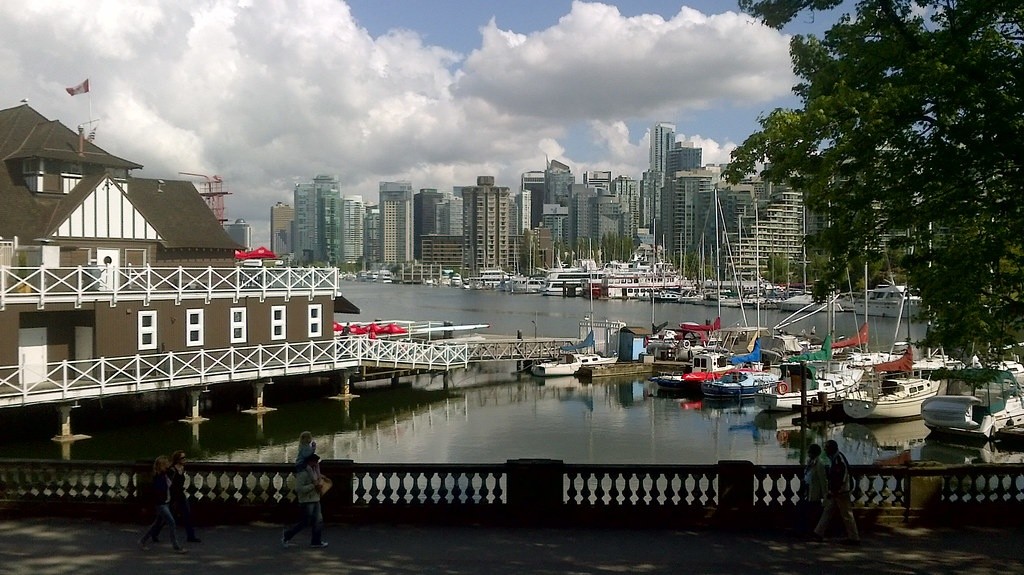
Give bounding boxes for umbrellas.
[332,321,410,339]
[234,247,278,269]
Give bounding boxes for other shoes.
[280,528,289,549]
[174,548,189,554]
[136,540,148,552]
[801,532,824,542]
[309,541,329,549]
[187,537,202,543]
[841,538,860,546]
[151,536,160,543]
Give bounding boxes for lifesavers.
[683,340,691,349]
[776,381,788,395]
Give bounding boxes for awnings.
[334,296,360,314]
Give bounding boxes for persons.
[150,450,203,543]
[281,429,330,549]
[340,322,351,354]
[792,440,860,545]
[137,454,190,553]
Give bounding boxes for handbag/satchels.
[312,474,333,497]
[842,476,856,495]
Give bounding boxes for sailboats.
[533,184,1024,440]
[506,239,544,294]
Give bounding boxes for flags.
[66,78,89,96]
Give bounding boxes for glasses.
[179,456,186,460]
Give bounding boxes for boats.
[462,267,513,290]
[339,268,461,288]
